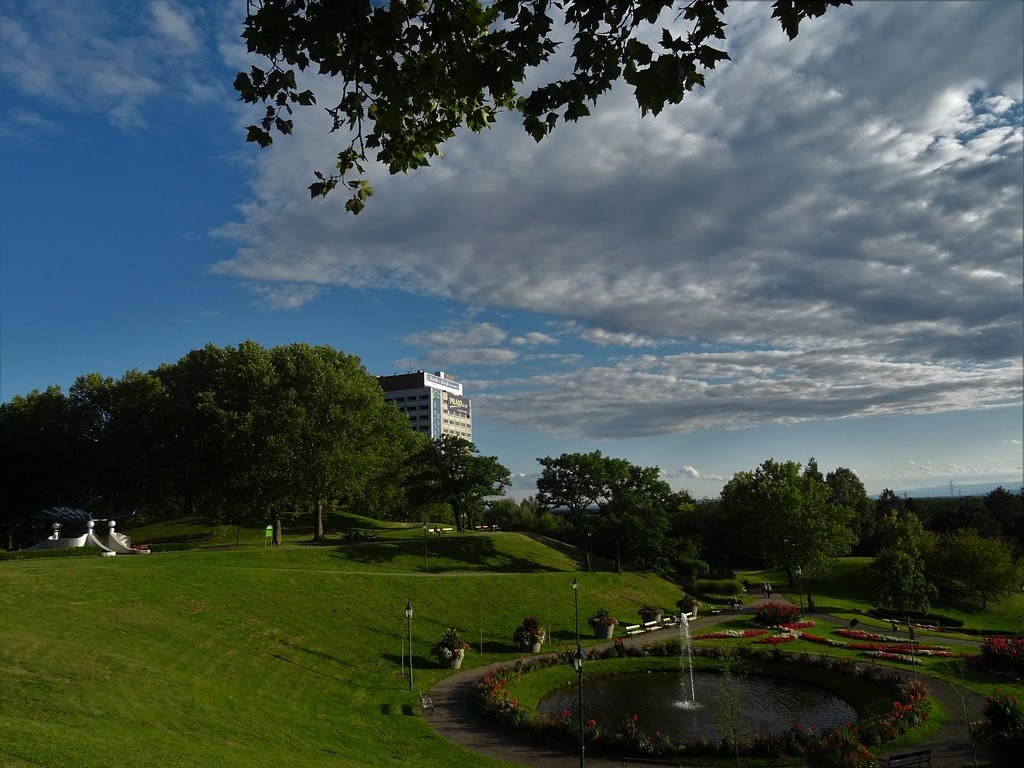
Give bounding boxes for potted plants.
[675,594,701,617]
[637,606,664,623]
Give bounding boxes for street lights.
[588,530,591,572]
[572,577,580,645]
[795,565,805,619]
[405,601,414,690]
[574,646,587,766]
[423,522,426,571]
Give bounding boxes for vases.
[594,624,613,638]
[439,655,462,669]
[522,641,540,652]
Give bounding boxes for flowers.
[430,627,470,660]
[588,608,619,626]
[514,616,545,645]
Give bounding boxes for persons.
[341,527,380,543]
[434,525,442,537]
[729,580,773,611]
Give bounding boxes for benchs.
[728,601,741,613]
[711,606,720,615]
[625,624,646,640]
[877,748,932,768]
[419,692,434,717]
[645,621,663,635]
[475,525,501,534]
[685,612,698,625]
[663,618,676,630]
[429,528,453,537]
